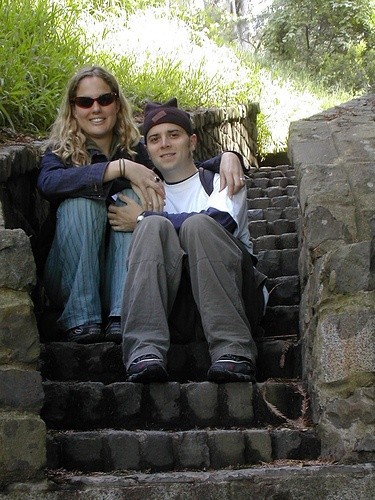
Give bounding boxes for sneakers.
[206,354,257,383]
[124,353,169,384]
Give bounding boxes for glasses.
[70,92,117,109]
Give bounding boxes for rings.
[240,177,245,181]
[155,177,160,183]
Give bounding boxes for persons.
[106,106,260,380]
[34,68,245,342]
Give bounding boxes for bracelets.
[118,158,126,178]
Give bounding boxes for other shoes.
[104,316,123,344]
[61,322,105,343]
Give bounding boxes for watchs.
[137,211,146,224]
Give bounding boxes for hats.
[143,97,194,143]
